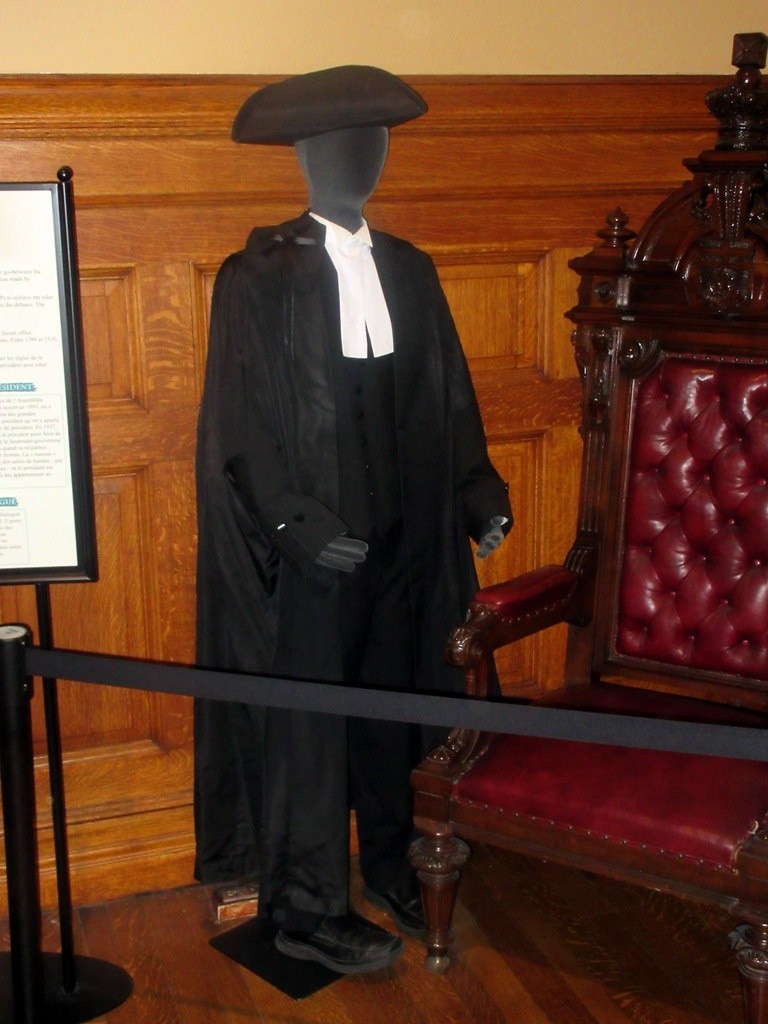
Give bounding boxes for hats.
[231,65,429,146]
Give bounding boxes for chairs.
[412,32,767,1024]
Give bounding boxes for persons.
[192,65,515,973]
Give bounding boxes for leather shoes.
[274,911,406,975]
[363,877,428,936]
